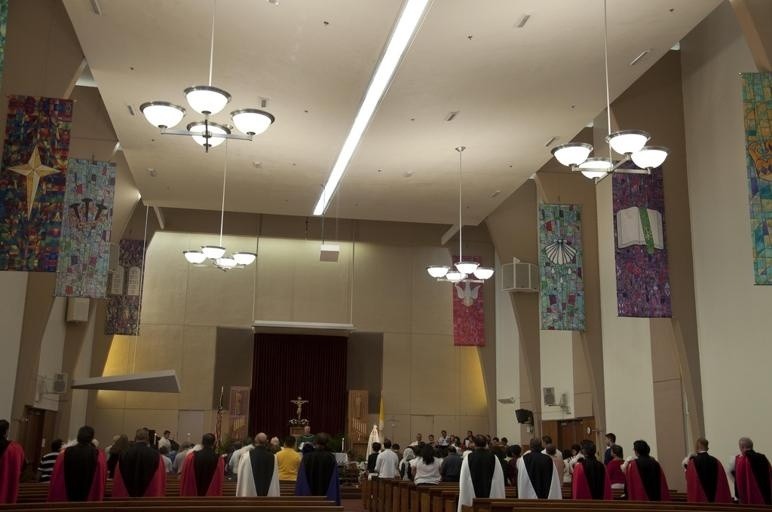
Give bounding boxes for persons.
[731,437,772,508]
[683,438,731,504]
[36,426,341,506]
[0,419,24,503]
[365,430,671,512]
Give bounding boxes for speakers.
[544,386,554,404]
[52,372,69,393]
[502,262,539,292]
[515,409,533,424]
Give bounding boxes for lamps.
[425,146,497,286]
[182,142,258,271]
[551,0,669,186]
[138,0,275,149]
